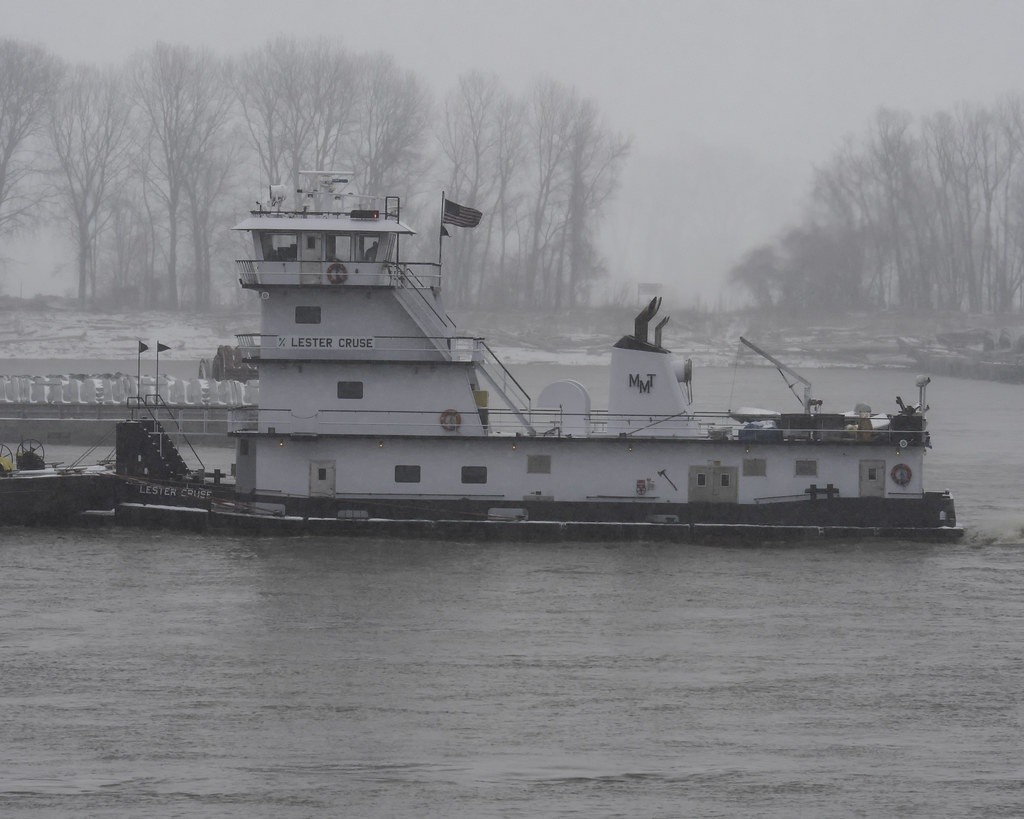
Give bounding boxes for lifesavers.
[890,463,912,486]
[440,409,462,432]
[327,264,348,284]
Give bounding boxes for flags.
[443,198,484,230]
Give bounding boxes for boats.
[0,431,117,528]
[117,169,967,550]
